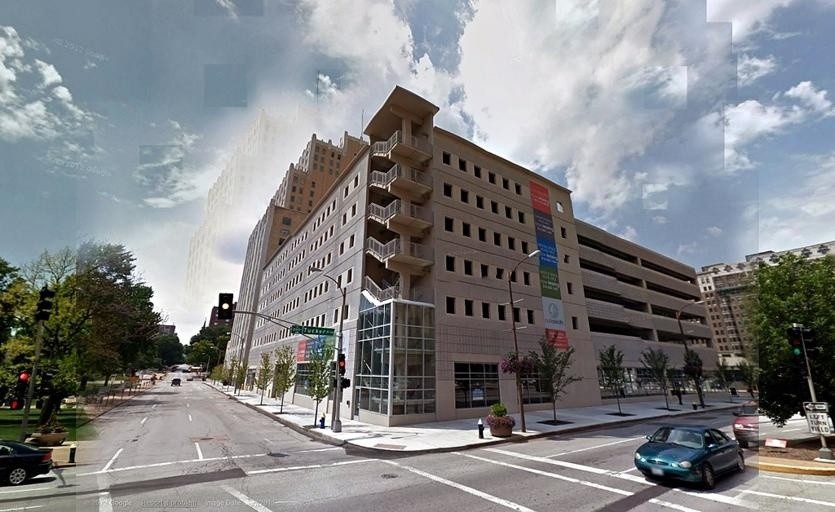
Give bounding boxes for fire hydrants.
[477,417,484,438]
[320,413,325,428]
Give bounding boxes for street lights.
[508,249,542,432]
[202,346,221,371]
[226,330,243,393]
[677,300,705,408]
[309,267,345,421]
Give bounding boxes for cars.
[732,400,760,447]
[187,376,193,381]
[634,424,745,491]
[162,363,192,372]
[171,377,181,386]
[0,439,53,485]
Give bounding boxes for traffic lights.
[38,371,53,402]
[787,328,804,357]
[35,284,55,323]
[334,378,350,388]
[802,325,820,360]
[218,293,233,320]
[10,370,30,410]
[339,354,345,374]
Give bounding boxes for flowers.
[486,414,516,429]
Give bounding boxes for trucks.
[192,362,207,372]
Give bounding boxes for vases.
[488,425,513,436]
